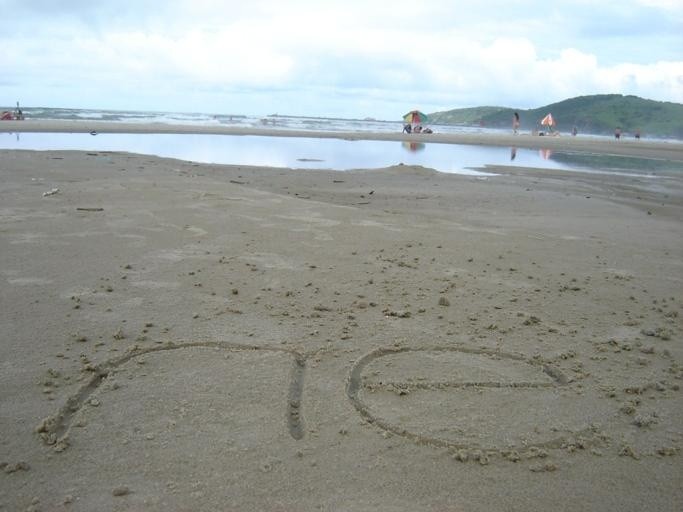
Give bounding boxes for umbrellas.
[403,111,427,124]
[541,112,557,130]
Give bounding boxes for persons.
[634,127,640,141]
[539,131,560,137]
[512,113,520,134]
[403,124,432,134]
[511,148,518,161]
[14,107,24,120]
[614,127,621,140]
